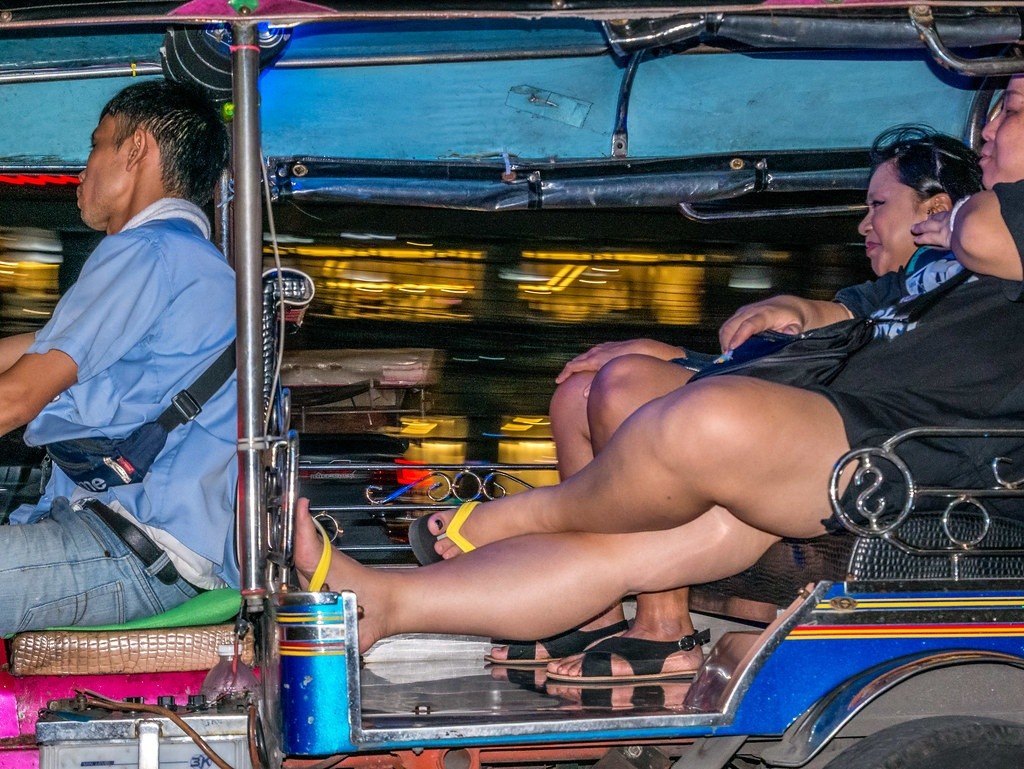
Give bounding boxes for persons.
[481,119,986,682]
[292,74,1024,655]
[1,80,239,638]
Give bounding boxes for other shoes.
[484,664,560,694]
[546,678,694,708]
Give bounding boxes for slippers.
[409,501,483,566]
[272,495,332,593]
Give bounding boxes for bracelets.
[949,194,971,233]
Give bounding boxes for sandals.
[546,628,710,683]
[484,619,629,664]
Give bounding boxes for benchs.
[10,265,316,676]
[689,426,1024,626]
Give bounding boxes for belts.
[85,499,179,585]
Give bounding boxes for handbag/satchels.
[686,318,875,391]
[45,422,169,492]
